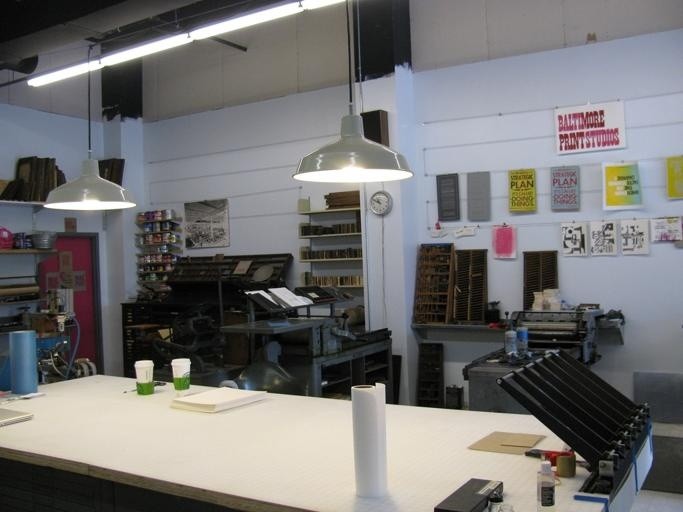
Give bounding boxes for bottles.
[535,460,555,511]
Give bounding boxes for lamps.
[23,0,346,90]
[293,0,414,185]
[42,42,141,216]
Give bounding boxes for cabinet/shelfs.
[296,193,363,290]
[285,337,395,405]
[0,178,45,336]
[134,216,182,294]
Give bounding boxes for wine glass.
[486,301,499,323]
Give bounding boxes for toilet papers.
[350,383,390,501]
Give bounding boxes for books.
[169,386,267,414]
[323,190,360,209]
[243,285,335,312]
[0,156,66,204]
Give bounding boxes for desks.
[0,372,655,512]
[467,347,581,414]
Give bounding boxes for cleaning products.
[505,324,528,359]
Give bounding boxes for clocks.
[368,189,393,217]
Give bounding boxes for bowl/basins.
[30,232,56,249]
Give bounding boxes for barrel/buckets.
[31,231,57,250]
[0,225,14,249]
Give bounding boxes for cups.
[556,456,575,477]
[302,246,356,259]
[302,222,354,236]
[133,359,154,395]
[504,326,529,354]
[170,357,191,392]
